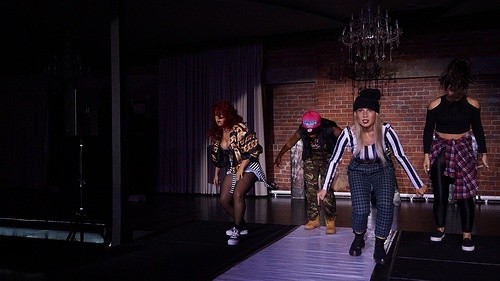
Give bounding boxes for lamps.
[337,0,402,62]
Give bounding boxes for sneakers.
[228,227,240,245]
[226,227,247,236]
[461,238,475,251]
[430,230,445,241]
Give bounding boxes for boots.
[349,230,367,256]
[373,236,387,265]
[304,213,320,229]
[325,219,336,234]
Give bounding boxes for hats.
[353,88,382,114]
[300,111,321,134]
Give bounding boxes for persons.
[422,56,490,251]
[317,89,427,264]
[208,100,272,247]
[275,110,344,235]
